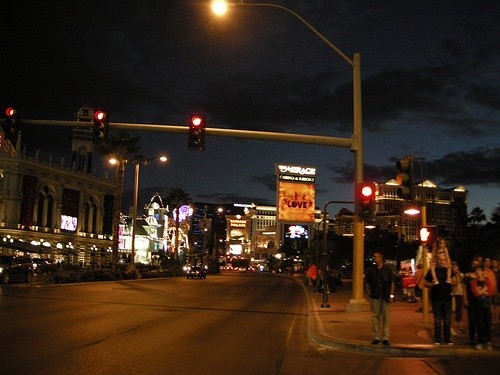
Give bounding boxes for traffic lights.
[357,182,375,222]
[418,225,438,245]
[187,114,207,152]
[396,157,415,200]
[92,107,110,145]
[2,104,20,140]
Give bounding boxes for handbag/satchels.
[415,285,422,296]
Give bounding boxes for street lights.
[209,0,372,314]
[109,156,167,266]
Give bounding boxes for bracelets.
[390,295,394,298]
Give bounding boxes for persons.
[366,252,395,345]
[400,262,424,303]
[424,237,500,349]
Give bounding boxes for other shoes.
[460,330,464,334]
[433,280,439,285]
[371,339,380,345]
[446,278,453,283]
[475,344,483,350]
[383,340,389,345]
[434,339,441,345]
[416,308,423,312]
[487,345,493,351]
[446,339,453,345]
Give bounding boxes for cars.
[33,258,53,272]
[186,265,207,279]
[0,254,35,284]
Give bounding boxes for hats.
[417,264,423,269]
